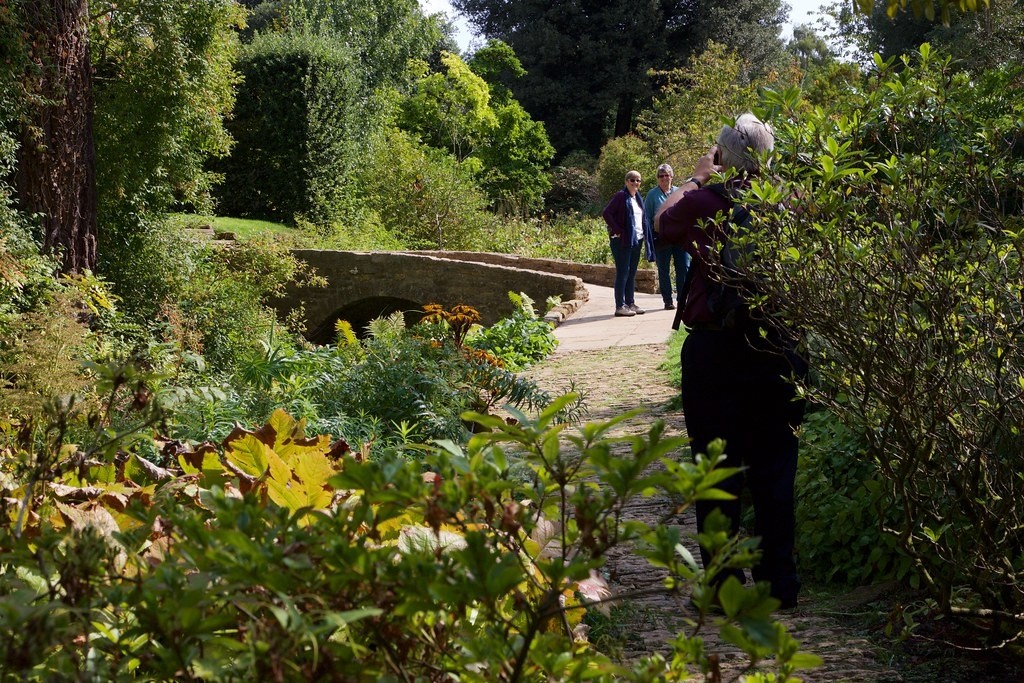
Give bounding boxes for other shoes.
[665,303,676,310]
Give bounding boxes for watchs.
[685,177,702,190]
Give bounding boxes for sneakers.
[629,303,645,314]
[615,304,636,316]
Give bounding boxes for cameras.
[714,152,719,165]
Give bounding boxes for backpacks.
[709,182,798,327]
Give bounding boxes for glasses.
[659,174,670,178]
[628,178,641,183]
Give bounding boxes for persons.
[603,170,646,315]
[645,162,690,311]
[654,114,810,612]
